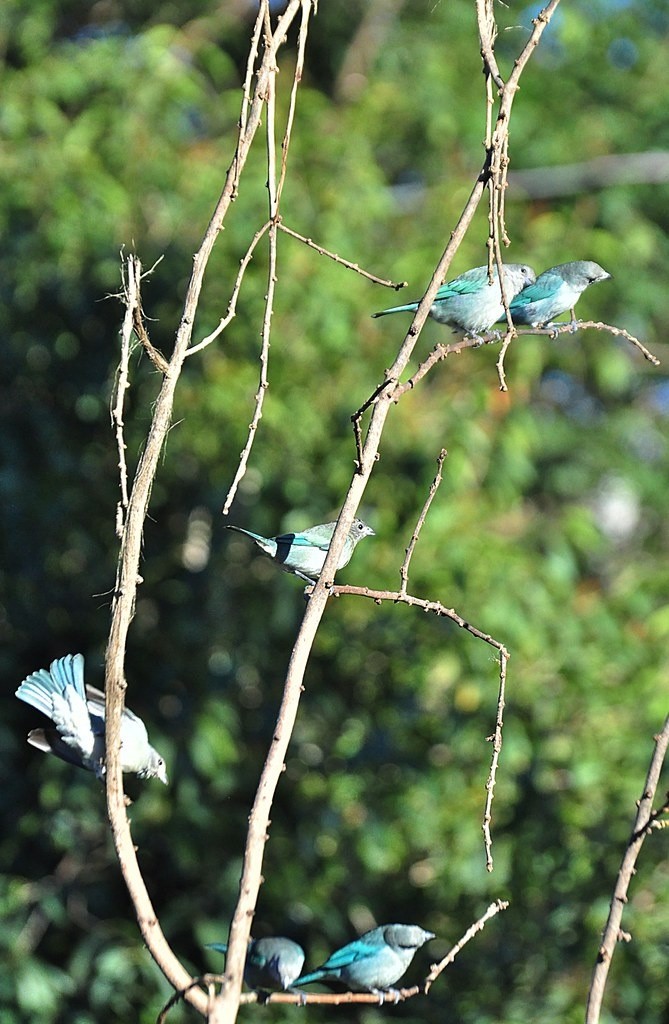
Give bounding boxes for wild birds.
[202,923,436,1006]
[222,518,376,584]
[16,653,168,786]
[370,260,612,347]
